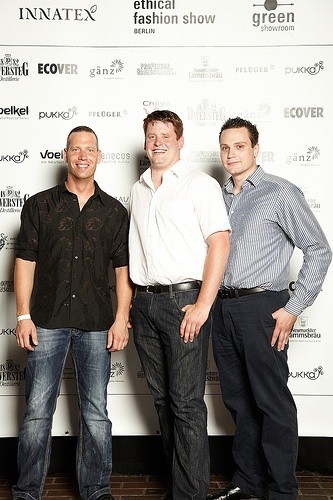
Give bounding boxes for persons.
[209,117,332,500]
[12,126,135,500]
[127,111,232,499]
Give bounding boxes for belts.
[138,281,201,295]
[217,287,266,299]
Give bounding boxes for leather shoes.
[207,486,268,500]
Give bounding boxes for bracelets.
[16,314,31,322]
